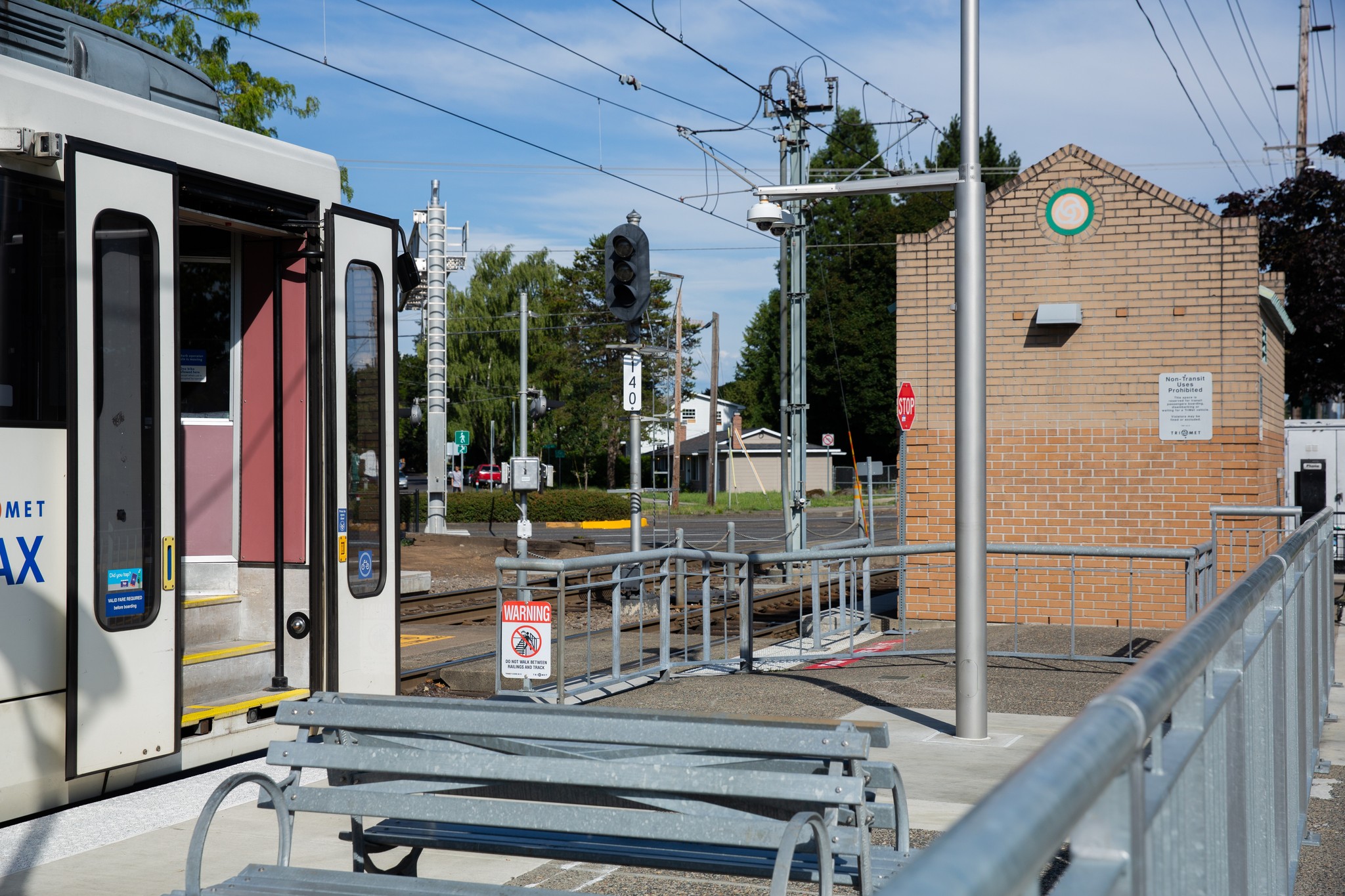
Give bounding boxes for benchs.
[307,689,923,896]
[147,699,873,896]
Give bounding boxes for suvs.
[472,464,502,487]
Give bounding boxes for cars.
[446,466,455,483]
[463,468,476,486]
[399,470,409,489]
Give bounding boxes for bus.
[0,0,422,831]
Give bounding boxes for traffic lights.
[603,223,650,320]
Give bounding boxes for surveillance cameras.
[770,209,795,237]
[746,200,783,232]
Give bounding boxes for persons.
[450,465,464,492]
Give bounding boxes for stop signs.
[897,382,916,431]
